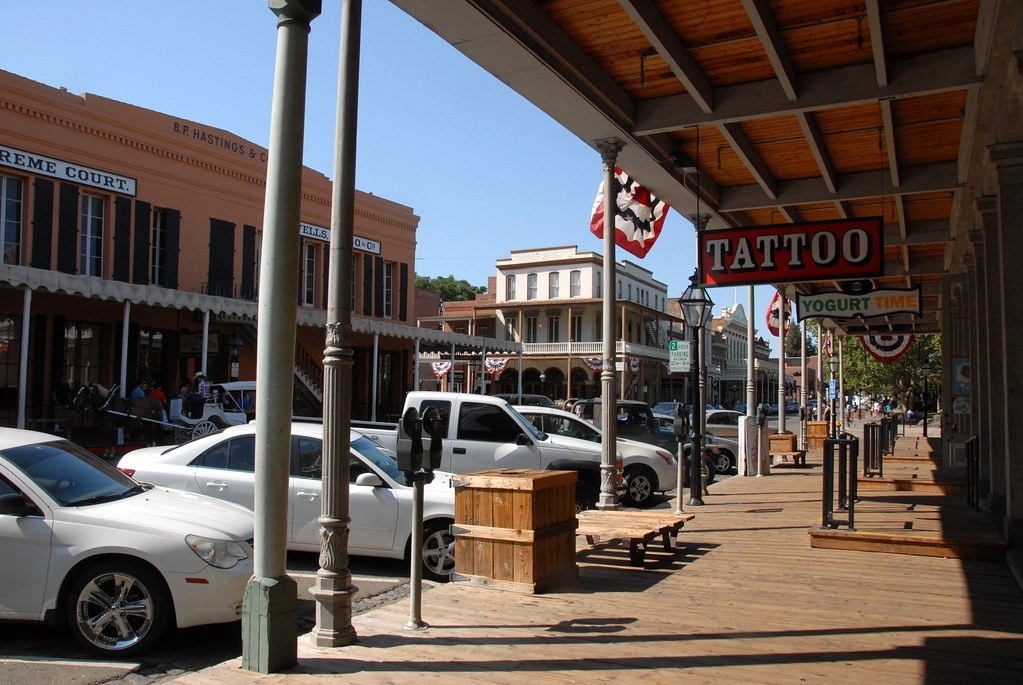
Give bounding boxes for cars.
[0,426,255,670]
[116,425,456,584]
[787,402,799,413]
[490,394,778,489]
[510,406,677,509]
[810,400,825,407]
[650,403,686,417]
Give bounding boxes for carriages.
[69,380,255,458]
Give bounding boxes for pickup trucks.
[244,389,630,513]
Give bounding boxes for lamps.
[672,159,701,175]
[838,320,847,326]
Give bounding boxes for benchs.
[192,396,225,418]
[28,419,74,443]
[577,509,696,564]
[769,449,809,467]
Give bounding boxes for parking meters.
[395,405,442,632]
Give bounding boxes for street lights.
[227,334,245,383]
[827,353,840,439]
[678,265,716,508]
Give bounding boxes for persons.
[845,401,858,428]
[870,400,913,419]
[50,373,71,432]
[808,402,831,422]
[125,372,255,441]
